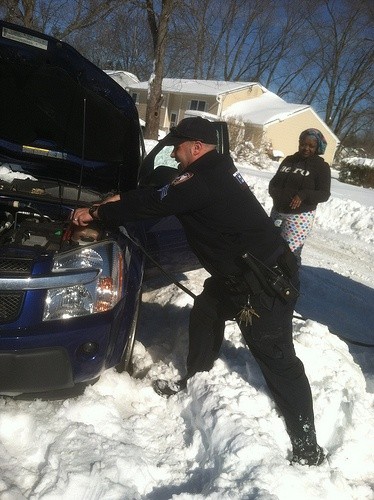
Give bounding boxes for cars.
[0,19,230,395]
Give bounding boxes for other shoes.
[289,445,329,467]
[154,378,187,398]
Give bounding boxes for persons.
[70,116,325,466]
[269,128,331,268]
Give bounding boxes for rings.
[72,217,79,221]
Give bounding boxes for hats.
[158,116,217,146]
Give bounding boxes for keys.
[237,306,260,327]
